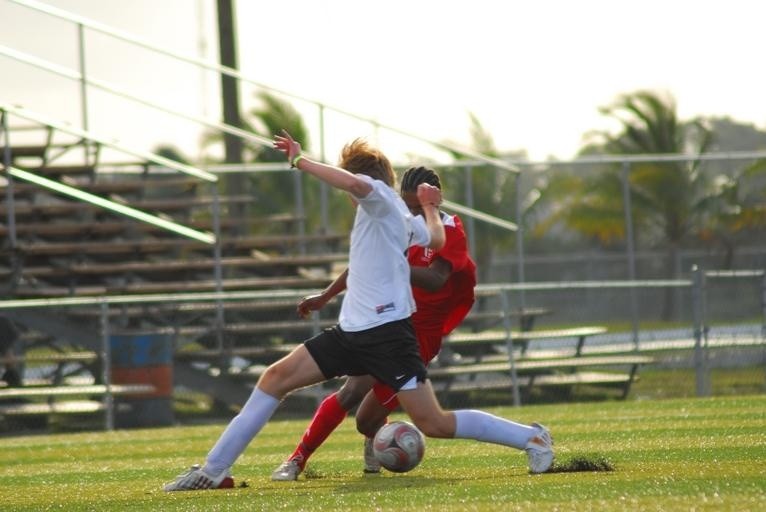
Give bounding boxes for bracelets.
[290,155,304,167]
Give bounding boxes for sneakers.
[526,422,553,474]
[271,460,303,481]
[364,416,387,473]
[161,466,238,490]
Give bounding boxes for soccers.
[373,419,424,472]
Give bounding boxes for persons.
[268,163,480,485]
[157,126,558,492]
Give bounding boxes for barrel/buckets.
[93,327,175,427]
